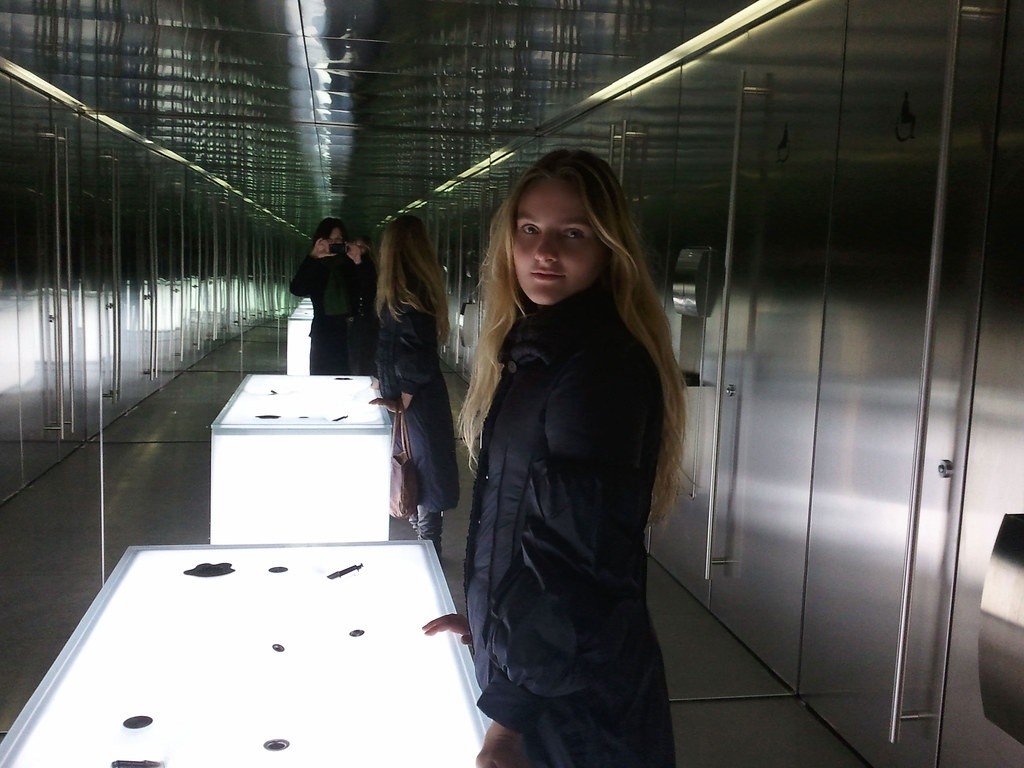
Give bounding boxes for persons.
[290,217,378,376]
[419,149,683,767]
[370,214,459,554]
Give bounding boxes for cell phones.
[328,243,348,254]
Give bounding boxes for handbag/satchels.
[389,399,421,518]
